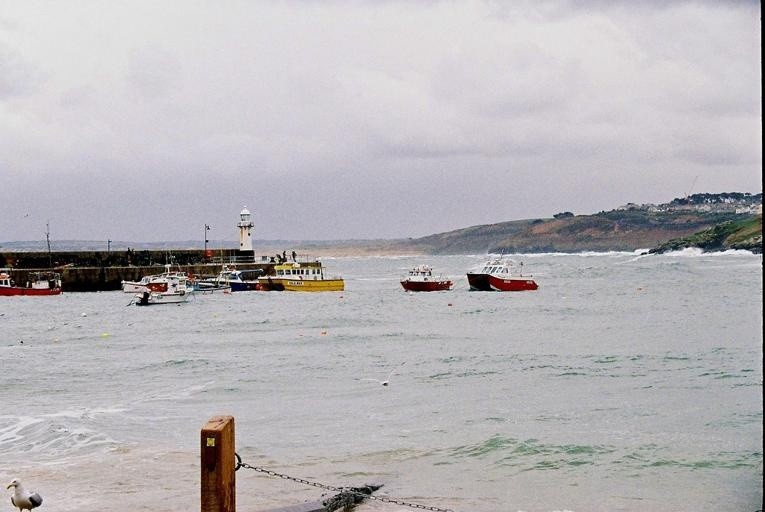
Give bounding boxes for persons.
[269,250,297,263]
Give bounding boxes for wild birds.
[353,360,407,388]
[6,477,44,512]
